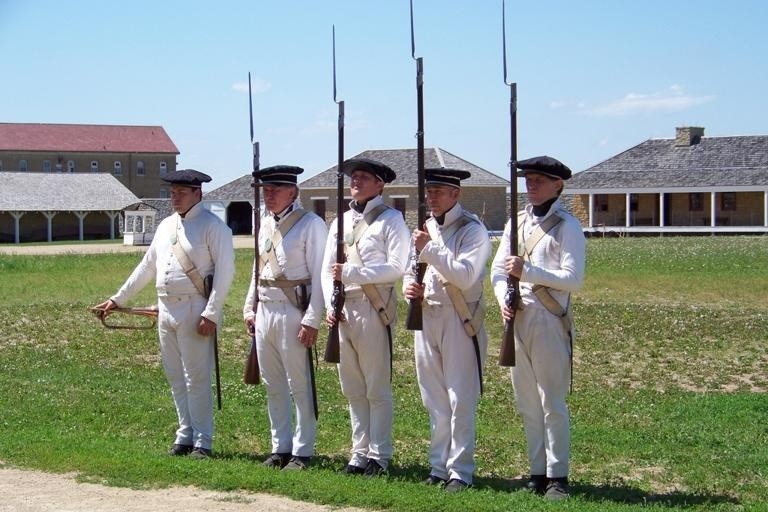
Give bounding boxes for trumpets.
[86,301,158,329]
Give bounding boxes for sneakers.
[345,458,390,481]
[528,475,572,500]
[263,453,310,471]
[422,476,472,493]
[168,444,212,459]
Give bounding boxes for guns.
[406,2,428,330]
[500,3,520,366]
[242,73,262,385]
[324,26,345,363]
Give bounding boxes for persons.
[323,157,414,479]
[489,155,587,498]
[244,166,331,469]
[95,169,237,458]
[401,166,492,489]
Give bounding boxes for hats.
[343,159,396,183]
[161,170,212,188]
[517,156,571,180]
[251,166,304,187]
[417,169,471,189]
